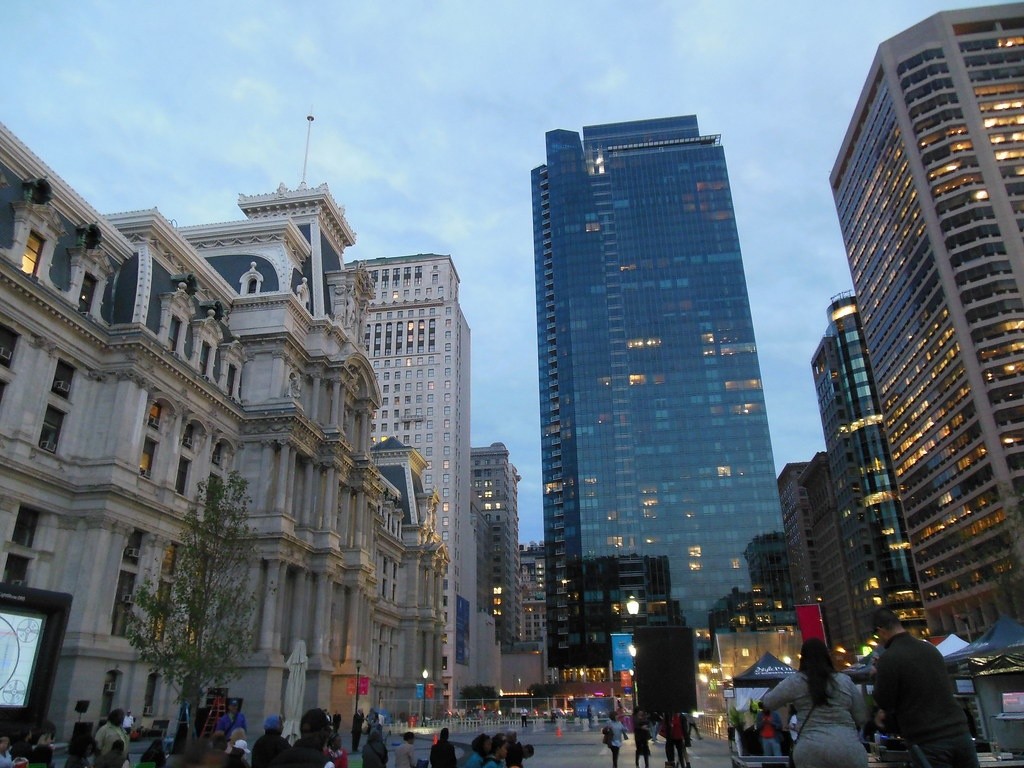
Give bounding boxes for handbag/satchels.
[789,741,796,768]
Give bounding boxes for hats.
[228,699,239,706]
[300,708,335,732]
[229,728,247,740]
[232,738,250,753]
[263,714,280,730]
[127,709,131,713]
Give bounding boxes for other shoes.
[698,737,703,741]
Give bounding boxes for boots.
[686,762,691,768]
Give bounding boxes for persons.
[361,732,388,768]
[141,740,166,768]
[521,705,528,728]
[587,705,594,729]
[756,635,871,767]
[863,607,979,768]
[365,708,394,752]
[181,708,347,768]
[395,732,418,768]
[351,707,365,752]
[93,740,132,768]
[95,708,130,759]
[166,689,198,755]
[430,728,458,768]
[216,699,247,741]
[64,735,93,768]
[460,729,534,768]
[0,725,57,768]
[606,700,702,768]
[551,711,556,723]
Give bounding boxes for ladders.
[197,696,226,742]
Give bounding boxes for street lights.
[421,669,428,727]
[355,658,362,714]
[626,596,639,729]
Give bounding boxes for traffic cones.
[557,724,562,736]
[432,734,438,746]
